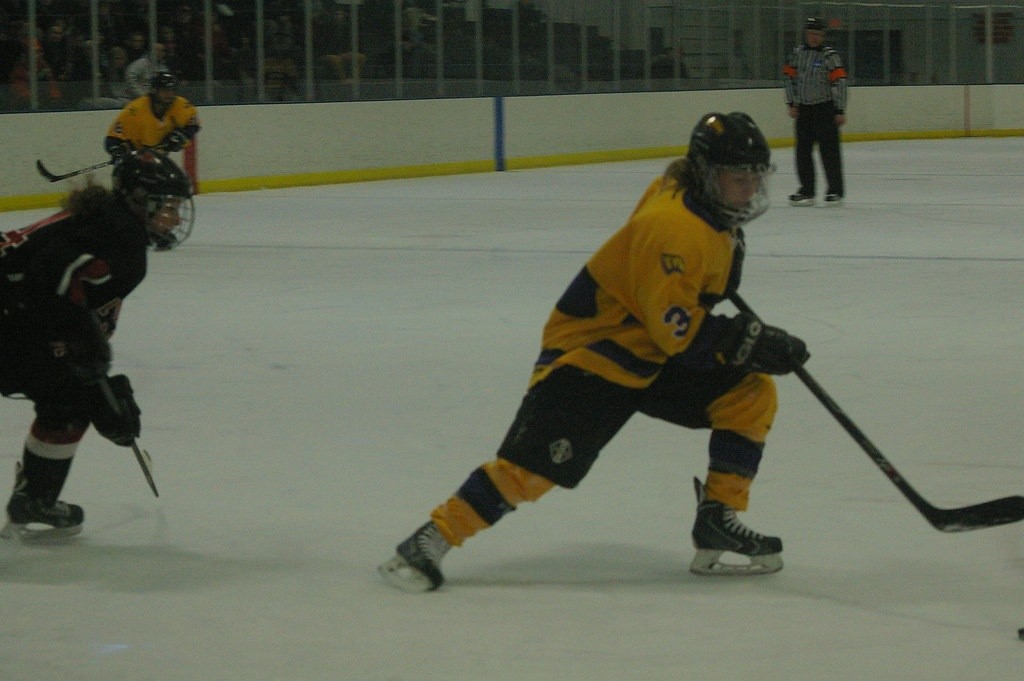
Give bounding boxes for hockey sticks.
[34,142,173,185]
[52,341,161,503]
[730,291,1024,534]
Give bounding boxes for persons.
[0,144,196,544]
[104,70,202,251]
[779,15,851,209]
[374,110,811,589]
[0,0,687,112]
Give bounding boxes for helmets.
[151,73,178,107]
[803,15,826,46]
[112,146,195,250]
[684,111,770,220]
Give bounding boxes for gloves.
[721,312,810,375]
[85,374,140,448]
[61,316,111,378]
[107,145,128,167]
[159,126,192,153]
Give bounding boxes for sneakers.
[824,191,844,205]
[378,519,451,595]
[690,478,784,575]
[0,463,84,542]
[788,191,817,207]
